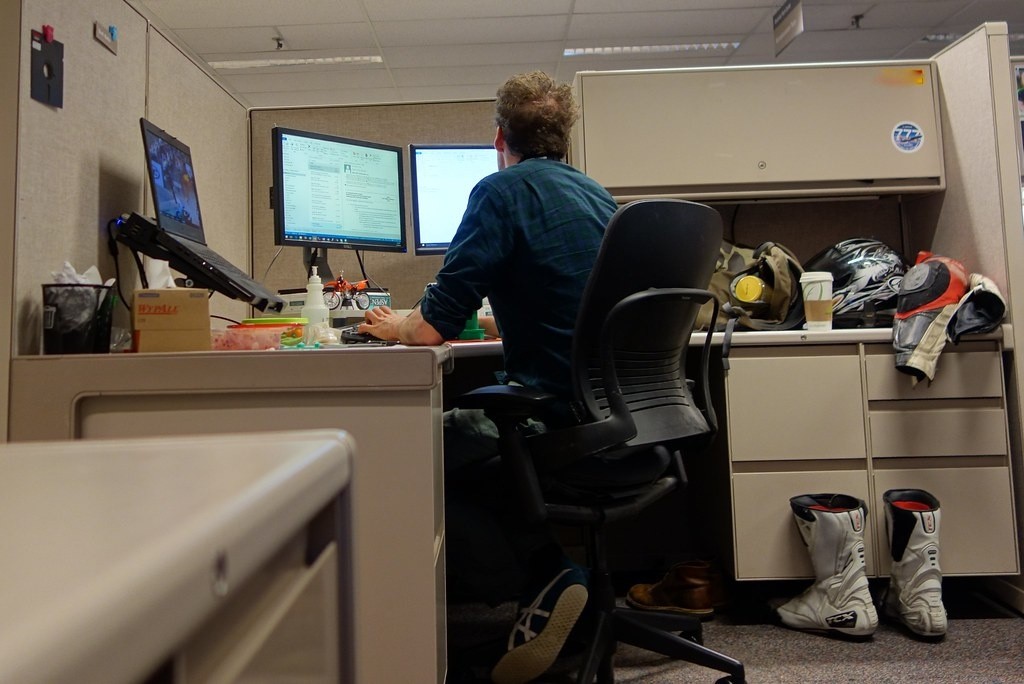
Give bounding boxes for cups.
[800,272,833,331]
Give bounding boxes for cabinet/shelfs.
[569,58,947,206]
[865,343,1021,578]
[724,346,875,581]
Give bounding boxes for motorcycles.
[323,269,370,310]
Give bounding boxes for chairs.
[465,199,744,684]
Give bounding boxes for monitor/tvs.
[270,127,407,293]
[406,143,499,255]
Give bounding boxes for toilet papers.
[142,253,176,289]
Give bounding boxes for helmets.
[808,237,904,326]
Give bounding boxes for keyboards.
[340,326,399,345]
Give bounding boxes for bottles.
[300,266,329,346]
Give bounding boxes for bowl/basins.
[241,317,309,349]
[225,324,285,349]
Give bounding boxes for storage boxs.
[131,288,211,351]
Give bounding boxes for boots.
[773,490,877,635]
[881,486,946,641]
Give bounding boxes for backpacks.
[690,235,807,332]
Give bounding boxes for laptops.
[139,116,289,311]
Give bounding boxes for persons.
[358,74,670,684]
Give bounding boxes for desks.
[14,326,1020,684]
[1,428,362,684]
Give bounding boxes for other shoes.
[624,562,715,621]
[492,566,588,684]
